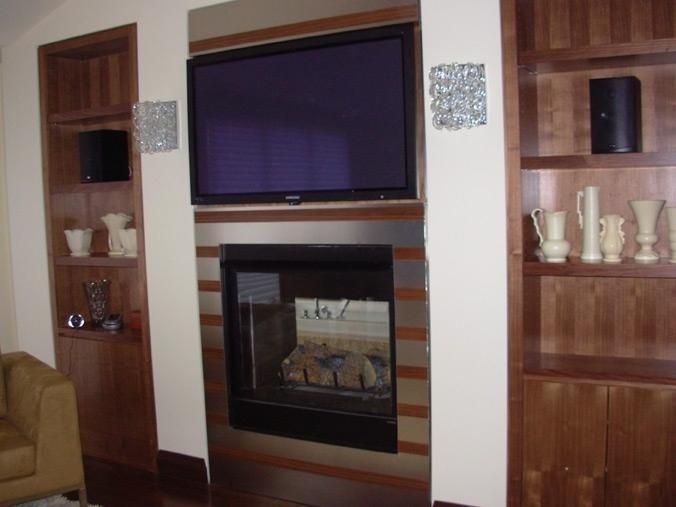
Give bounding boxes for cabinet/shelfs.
[497,0,675,507]
[35,21,157,469]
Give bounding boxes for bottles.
[575,186,602,263]
[599,214,626,263]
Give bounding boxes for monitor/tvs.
[187,21,417,204]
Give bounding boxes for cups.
[100,211,133,256]
[628,199,664,264]
[63,228,94,257]
[118,229,137,256]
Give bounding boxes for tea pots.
[530,207,572,263]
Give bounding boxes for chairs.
[0,351,86,506]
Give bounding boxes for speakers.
[78,130,129,183]
[590,76,641,154]
